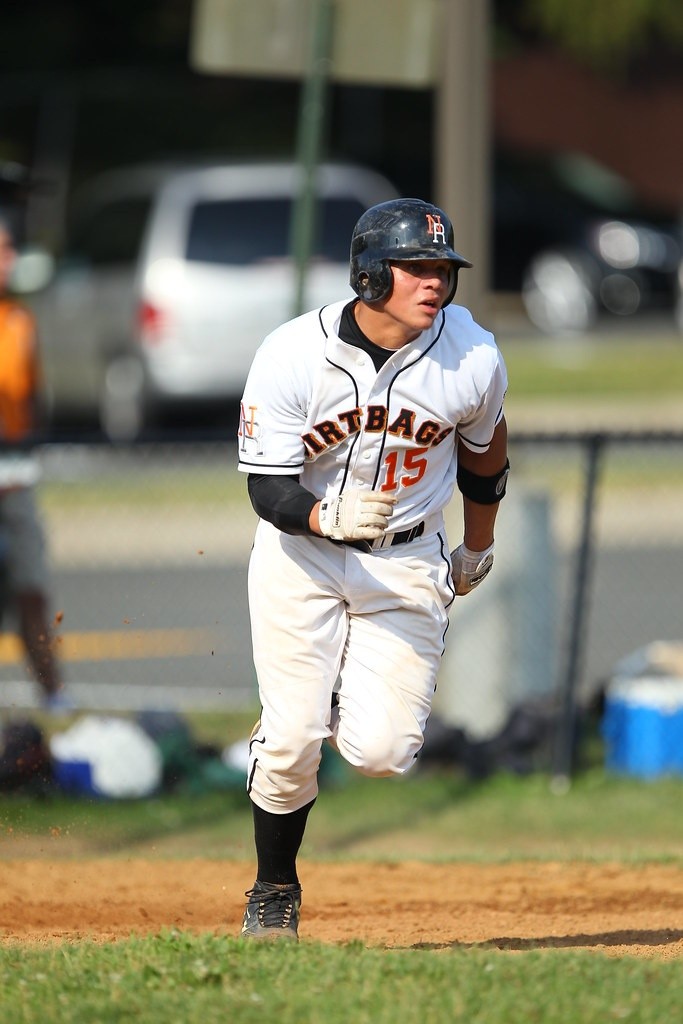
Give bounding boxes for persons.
[236,198,510,951]
[0,216,74,783]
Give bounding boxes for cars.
[137,165,404,435]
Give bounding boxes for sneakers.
[241,878,301,945]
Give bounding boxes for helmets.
[349,198,474,309]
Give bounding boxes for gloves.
[450,541,495,596]
[317,487,401,541]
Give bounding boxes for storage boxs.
[604,674,682,778]
